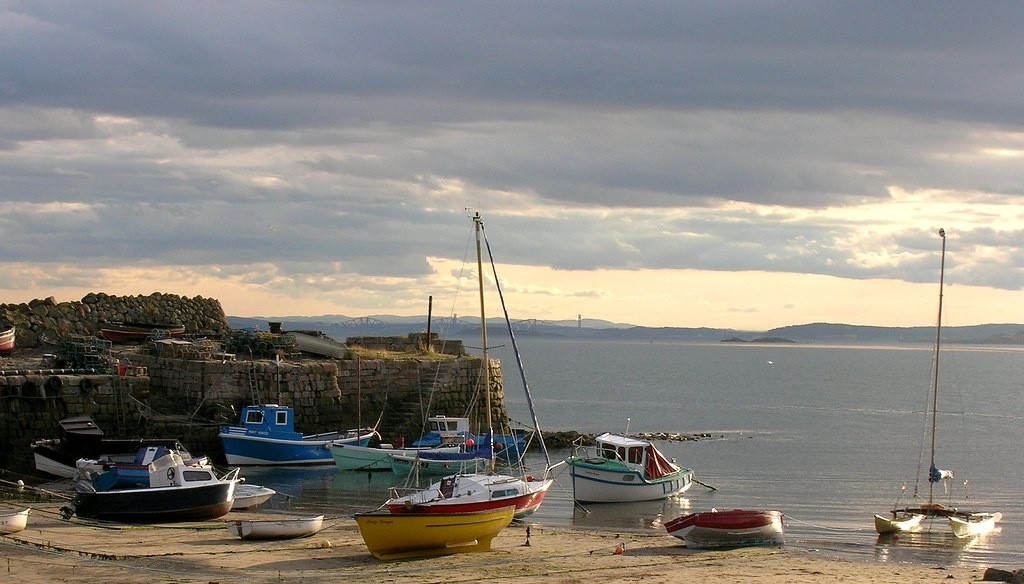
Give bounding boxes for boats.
[327,415,524,478]
[97,319,185,343]
[890,227,970,519]
[287,331,356,359]
[385,205,556,525]
[662,507,786,550]
[0,326,15,357]
[353,504,517,561]
[226,514,325,540]
[874,483,926,535]
[219,354,376,467]
[1,508,31,535]
[565,417,694,505]
[948,479,996,540]
[30,415,276,523]
